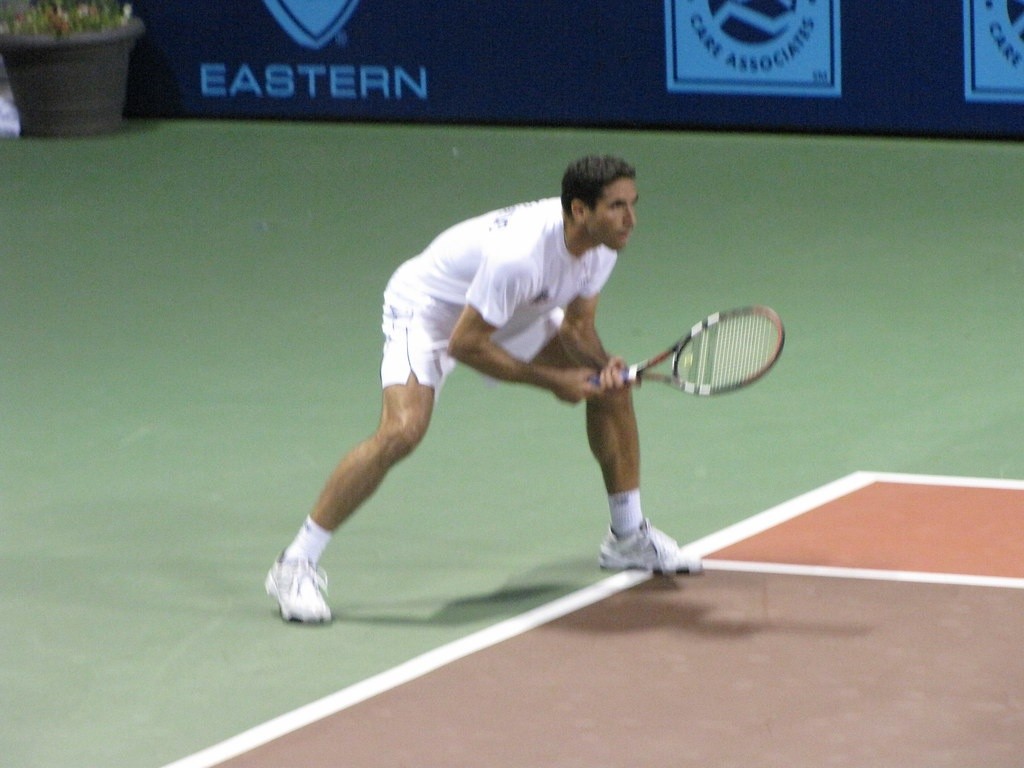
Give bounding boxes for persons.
[265,153,706,625]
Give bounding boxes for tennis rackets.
[585,304,786,396]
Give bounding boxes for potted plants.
[0,1,144,139]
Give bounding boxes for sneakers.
[265,547,332,623]
[599,518,703,574]
